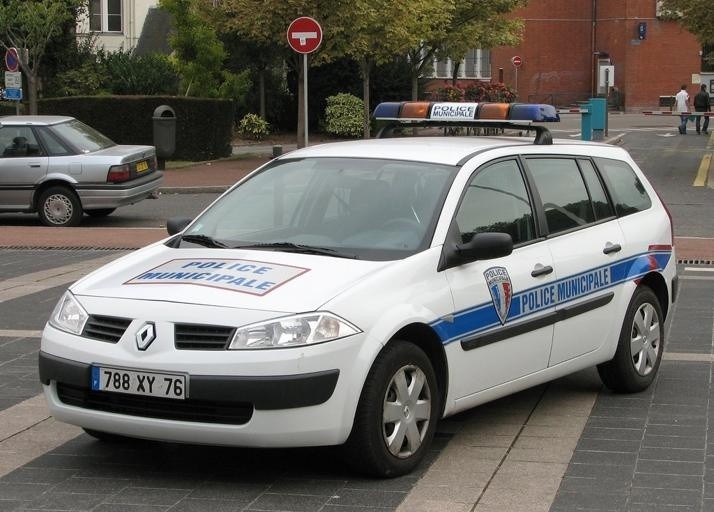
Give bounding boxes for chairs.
[325,179,396,245]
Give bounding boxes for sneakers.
[678,126,709,136]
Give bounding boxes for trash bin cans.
[151,105,176,170]
[611,87,620,111]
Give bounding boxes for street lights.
[592,52,609,97]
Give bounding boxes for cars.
[1,115,163,226]
[38,101,680,477]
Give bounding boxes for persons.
[3,132,40,158]
[693,83,712,135]
[673,83,692,135]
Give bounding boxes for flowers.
[441,82,520,105]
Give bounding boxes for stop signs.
[512,56,523,68]
[286,16,324,56]
